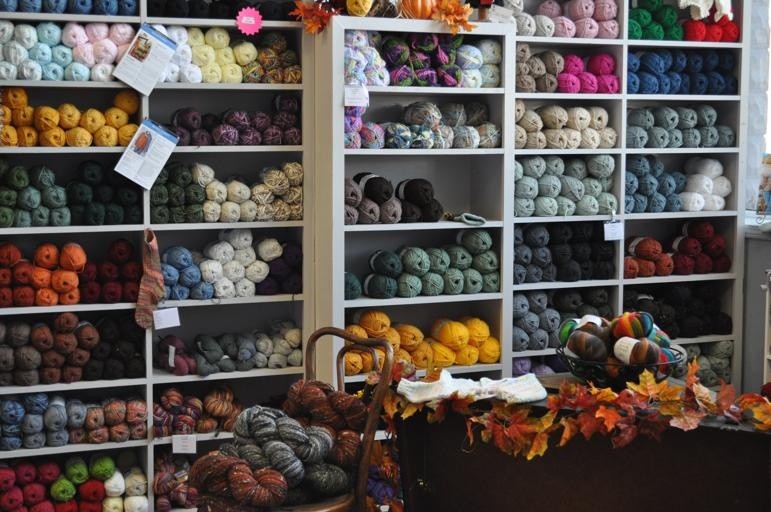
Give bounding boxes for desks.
[388,386,771,512]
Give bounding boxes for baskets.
[554,343,684,387]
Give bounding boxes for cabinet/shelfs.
[0,1,323,512]
[337,18,507,400]
[480,0,740,394]
